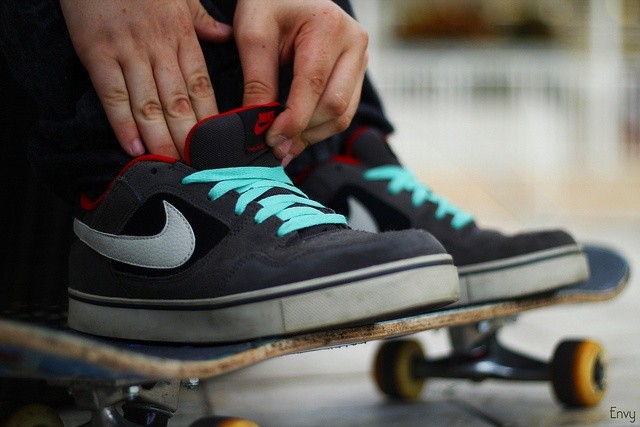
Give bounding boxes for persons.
[0,1,590,342]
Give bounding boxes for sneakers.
[62,100,463,344]
[301,127,590,307]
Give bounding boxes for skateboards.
[0,244,630,427]
